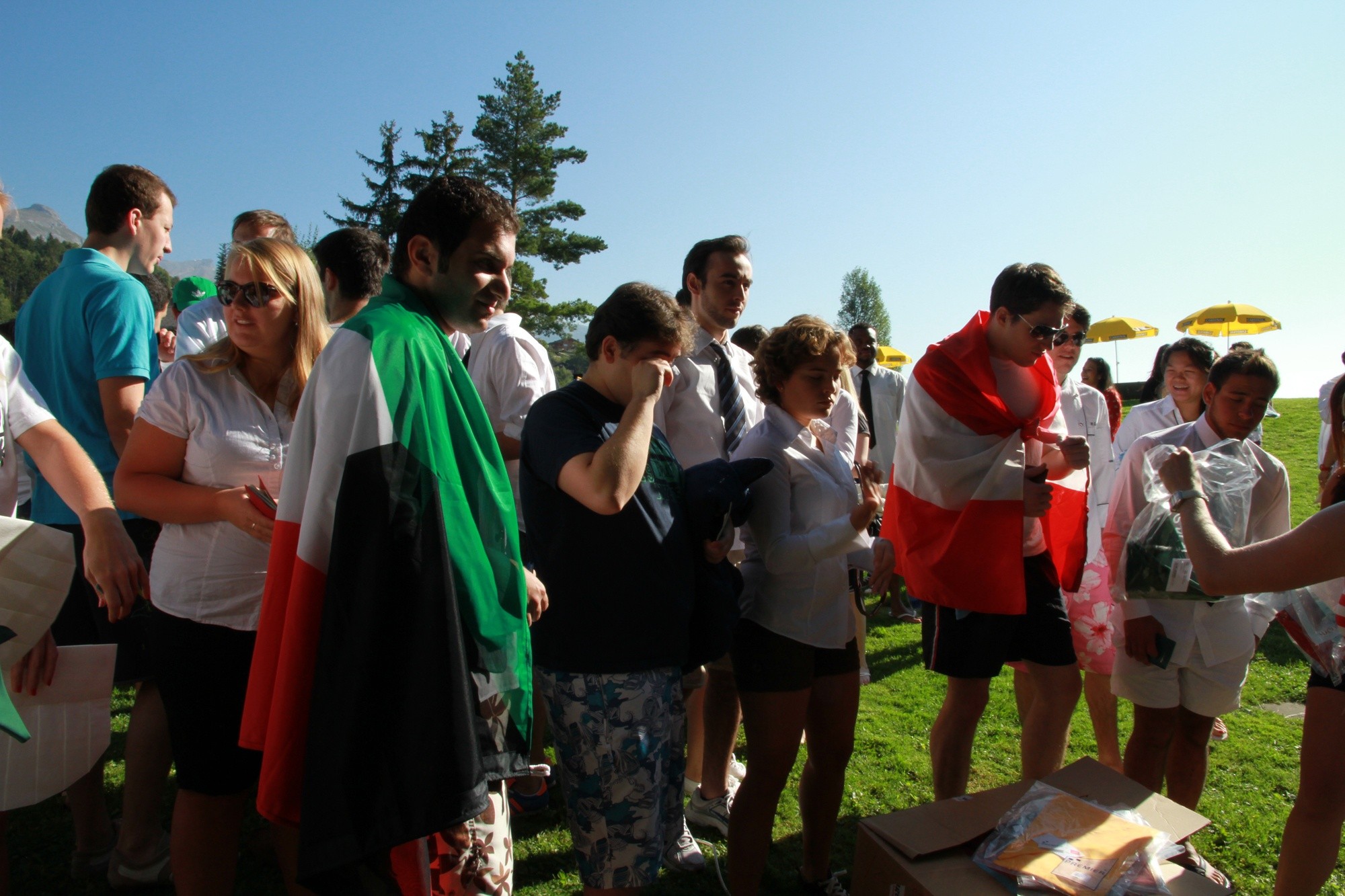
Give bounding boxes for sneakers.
[662,814,707,876]
[504,763,552,819]
[798,865,850,896]
[684,787,735,837]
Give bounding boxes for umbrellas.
[1084,315,1159,383]
[1176,300,1282,353]
[874,344,912,368]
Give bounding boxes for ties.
[859,370,876,449]
[711,341,747,454]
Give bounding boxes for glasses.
[218,273,298,312]
[1019,314,1070,340]
[1053,328,1086,346]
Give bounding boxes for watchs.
[1168,490,1209,512]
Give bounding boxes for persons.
[174,209,299,360]
[0,177,151,696]
[1158,353,1345,896]
[159,276,216,371]
[14,164,173,887]
[238,173,736,896]
[878,262,1291,887]
[113,235,331,896]
[131,271,176,361]
[0,318,16,349]
[654,230,895,896]
[1082,357,1122,444]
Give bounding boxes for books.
[245,474,277,521]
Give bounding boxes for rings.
[251,523,256,528]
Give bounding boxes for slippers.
[1168,840,1237,896]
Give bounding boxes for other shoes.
[683,771,741,797]
[726,752,747,781]
[68,832,121,881]
[105,830,176,890]
[1209,717,1228,742]
